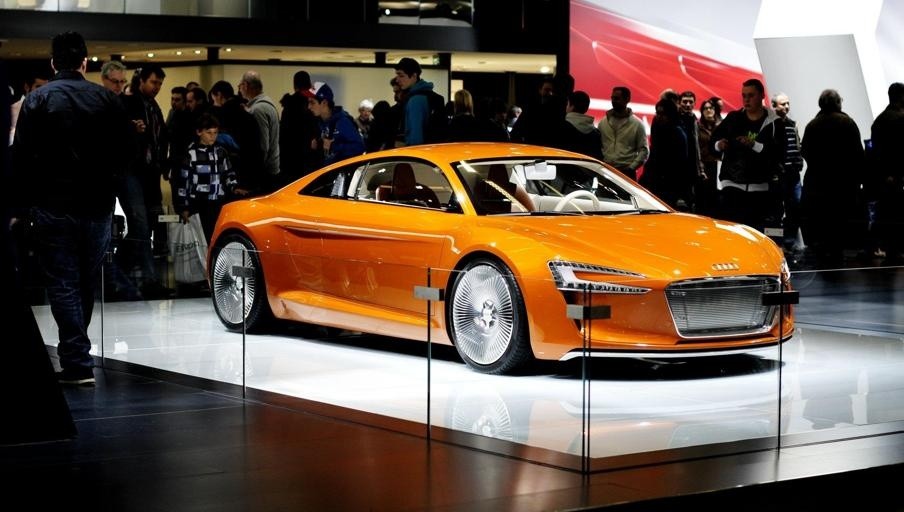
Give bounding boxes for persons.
[307,57,480,198]
[491,73,722,210]
[710,79,902,269]
[2,34,132,383]
[97,59,324,303]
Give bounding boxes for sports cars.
[202,141,794,376]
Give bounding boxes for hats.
[300,81,333,100]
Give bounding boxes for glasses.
[106,77,127,84]
[705,106,715,110]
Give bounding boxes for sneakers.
[785,239,805,254]
[55,365,96,384]
[107,282,212,300]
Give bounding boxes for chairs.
[376,161,441,209]
[487,165,538,213]
